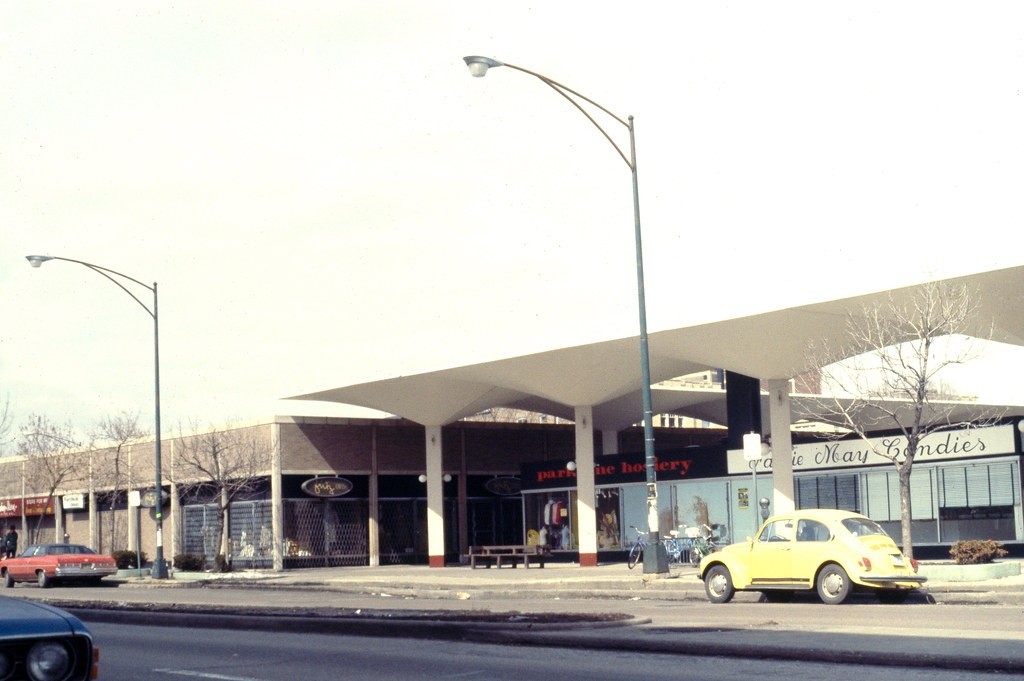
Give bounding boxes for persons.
[3,524,18,558]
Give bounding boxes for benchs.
[468,545,546,570]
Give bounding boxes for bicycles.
[625,524,684,570]
[689,524,718,567]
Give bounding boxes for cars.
[697,507,928,605]
[0,544,119,588]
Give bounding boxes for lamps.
[566,461,576,471]
[418,474,427,483]
[442,474,452,483]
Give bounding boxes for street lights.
[462,54,670,573]
[26,254,169,580]
